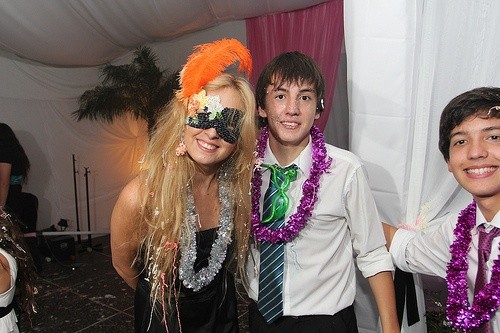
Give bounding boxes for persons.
[381,87,500,333]
[239,50,400,333]
[110,40,257,333]
[0,123,42,333]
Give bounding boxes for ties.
[257,164,300,324]
[472,224,500,333]
